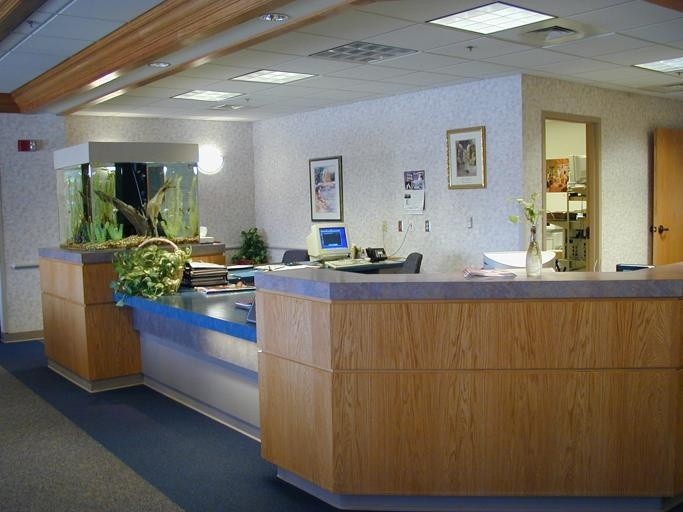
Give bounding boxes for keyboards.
[325,258,372,269]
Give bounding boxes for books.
[184,261,229,287]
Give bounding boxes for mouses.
[370,258,379,262]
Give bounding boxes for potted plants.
[230,227,268,266]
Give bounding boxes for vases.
[525,227,543,280]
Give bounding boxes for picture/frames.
[307,157,345,224]
[444,126,487,190]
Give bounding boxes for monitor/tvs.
[307,223,352,262]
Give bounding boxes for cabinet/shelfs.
[547,186,591,272]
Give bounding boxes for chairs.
[278,249,308,263]
[392,252,422,274]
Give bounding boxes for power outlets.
[382,224,388,232]
[407,220,414,231]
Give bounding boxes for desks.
[224,254,398,282]
[112,283,260,445]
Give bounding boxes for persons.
[458,141,473,173]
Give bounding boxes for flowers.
[510,192,545,227]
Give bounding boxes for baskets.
[120,237,184,296]
[565,238,587,259]
